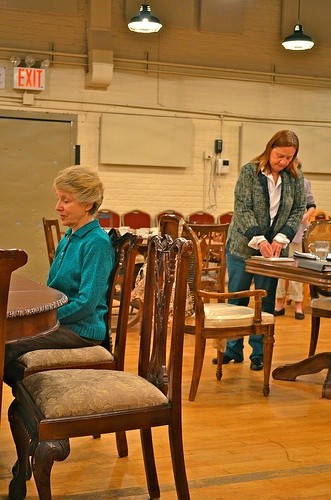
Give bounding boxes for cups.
[101,226,160,237]
[308,240,329,261]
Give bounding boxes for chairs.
[0,210,275,500]
[302,211,331,358]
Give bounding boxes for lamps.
[10,55,52,69]
[128,0,164,33]
[282,0,315,50]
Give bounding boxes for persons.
[4,165,115,386]
[274,178,316,320]
[212,130,307,370]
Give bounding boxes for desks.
[5,275,66,343]
[244,256,331,399]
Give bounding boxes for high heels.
[212,353,244,364]
[250,358,264,370]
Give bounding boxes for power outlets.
[205,152,211,159]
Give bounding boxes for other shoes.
[295,311,305,320]
[274,307,285,316]
